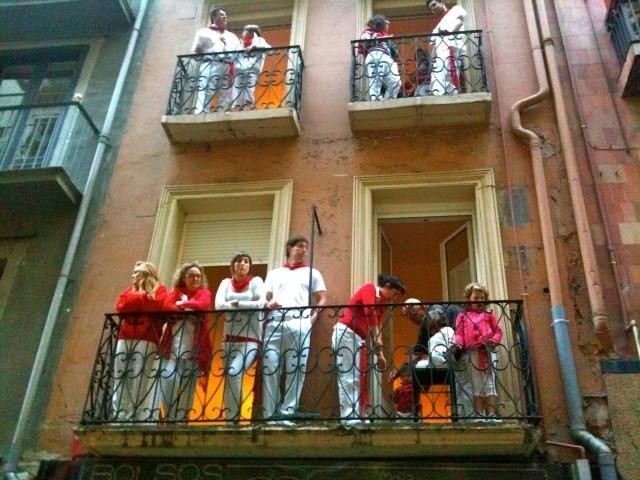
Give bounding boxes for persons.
[161,262,212,425]
[402,1,467,100]
[332,273,404,427]
[357,13,401,102]
[231,25,271,111]
[454,282,502,425]
[112,262,166,425]
[216,254,267,426]
[193,7,241,113]
[390,296,472,427]
[264,236,328,427]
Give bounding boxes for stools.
[412,367,458,422]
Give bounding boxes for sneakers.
[473,412,503,422]
